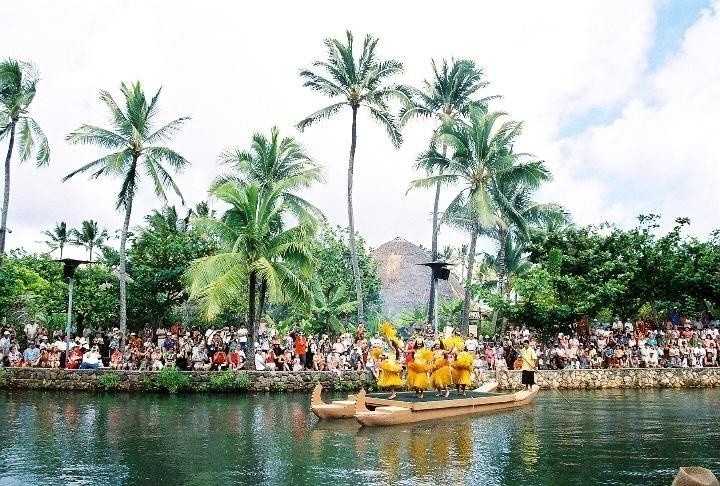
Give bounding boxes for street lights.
[48,258,96,368]
[416,259,455,340]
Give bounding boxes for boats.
[311,380,540,428]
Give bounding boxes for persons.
[494,353,511,390]
[450,311,536,381]
[372,320,475,398]
[186,319,383,372]
[532,314,720,370]
[0,318,194,371]
[517,339,538,391]
